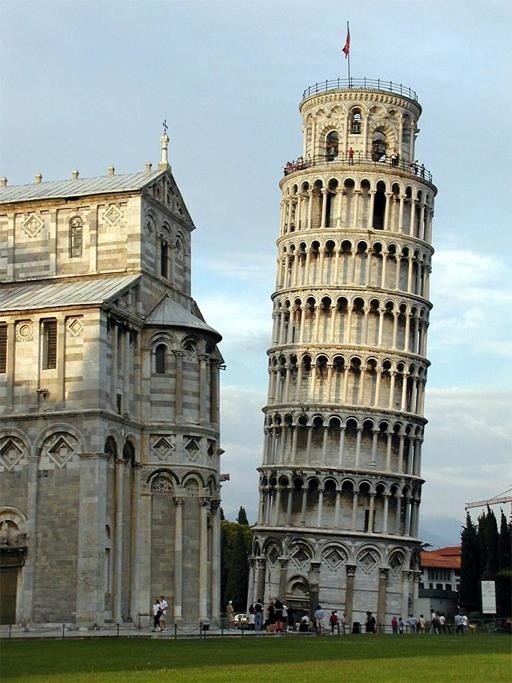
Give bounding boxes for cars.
[221,613,247,627]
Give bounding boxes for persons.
[281,155,307,175]
[391,615,397,633]
[414,159,419,174]
[390,149,397,166]
[152,599,161,632]
[227,599,235,628]
[160,594,169,631]
[348,146,355,165]
[246,598,376,633]
[416,614,426,633]
[398,616,403,633]
[405,614,417,632]
[427,607,468,633]
[420,162,425,178]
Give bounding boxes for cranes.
[465,489,512,524]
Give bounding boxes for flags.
[342,30,350,58]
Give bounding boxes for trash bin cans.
[353,621,360,634]
[220,613,229,629]
